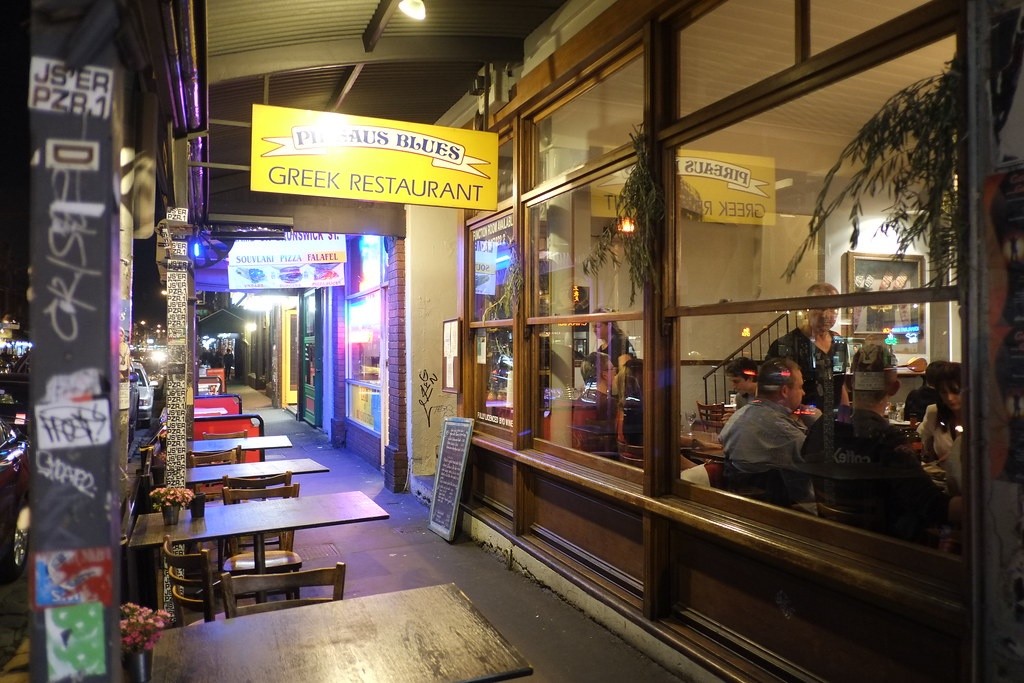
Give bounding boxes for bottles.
[487,381,497,415]
[497,381,506,418]
[905,414,924,464]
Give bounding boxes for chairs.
[162,432,347,629]
[803,454,887,533]
[695,400,725,432]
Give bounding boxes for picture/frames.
[847,250,925,340]
[845,336,866,369]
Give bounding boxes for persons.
[717,356,822,510]
[767,283,846,414]
[800,345,962,551]
[616,360,722,471]
[590,307,637,398]
[902,360,950,422]
[721,357,758,427]
[201,347,224,368]
[572,352,618,460]
[223,349,233,380]
[118,328,129,381]
[917,363,963,467]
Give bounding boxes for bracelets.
[692,438,697,449]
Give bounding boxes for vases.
[161,504,180,525]
[124,648,153,683]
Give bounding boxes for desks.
[132,434,534,683]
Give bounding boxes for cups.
[800,405,816,415]
[730,394,736,405]
[151,466,164,484]
[680,425,685,437]
[189,492,206,518]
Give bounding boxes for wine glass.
[685,410,696,435]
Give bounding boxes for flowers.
[120,602,169,655]
[150,486,195,511]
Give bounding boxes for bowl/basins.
[925,467,946,481]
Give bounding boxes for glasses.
[939,387,961,397]
